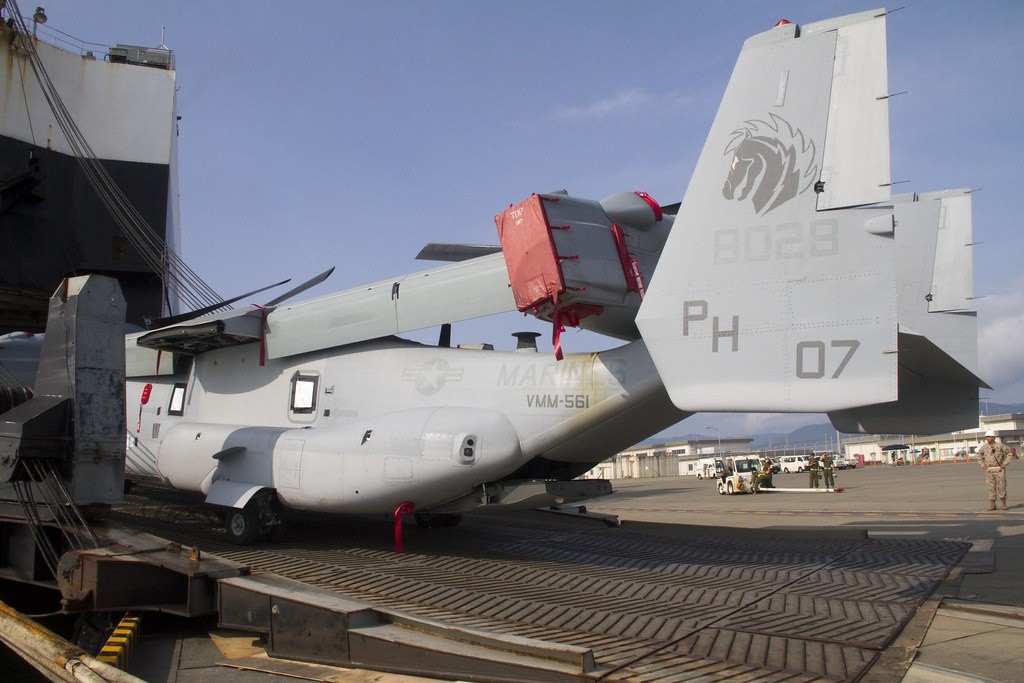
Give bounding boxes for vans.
[694,455,858,480]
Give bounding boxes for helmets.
[985,431,996,436]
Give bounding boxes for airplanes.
[0,8,994,548]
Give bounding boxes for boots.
[988,500,997,511]
[1000,499,1008,510]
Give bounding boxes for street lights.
[705,427,721,459]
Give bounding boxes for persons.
[978,431,1013,511]
[820,452,835,488]
[749,458,773,494]
[809,454,819,489]
